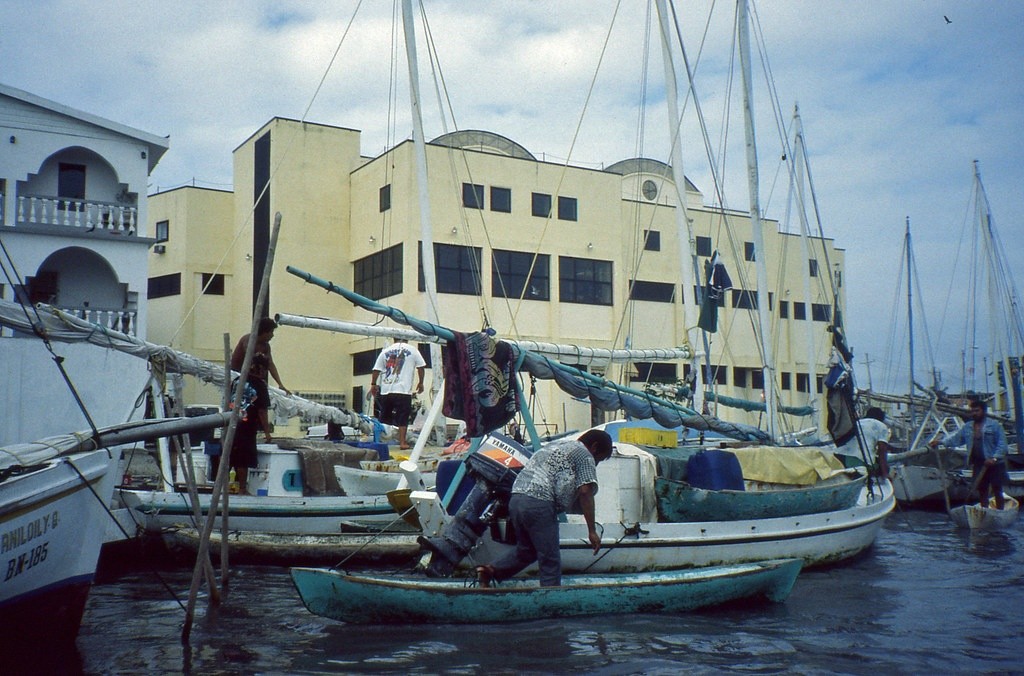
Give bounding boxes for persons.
[229,318,292,495]
[834,407,892,478]
[371,338,427,450]
[928,400,1009,511]
[477,429,613,588]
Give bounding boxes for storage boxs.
[566,450,658,523]
[245,444,388,497]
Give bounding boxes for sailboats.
[288,0,895,625]
[886,215,959,509]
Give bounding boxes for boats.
[946,491,1020,531]
[327,459,441,494]
[159,524,420,562]
[1,444,123,676]
[953,159,1024,505]
[118,485,417,534]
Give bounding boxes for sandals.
[400,446,412,450]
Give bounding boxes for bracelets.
[371,383,376,385]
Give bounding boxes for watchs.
[994,458,998,462]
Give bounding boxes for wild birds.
[943,14,954,25]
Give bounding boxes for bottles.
[230,467,236,483]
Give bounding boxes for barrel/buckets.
[176,456,208,486]
